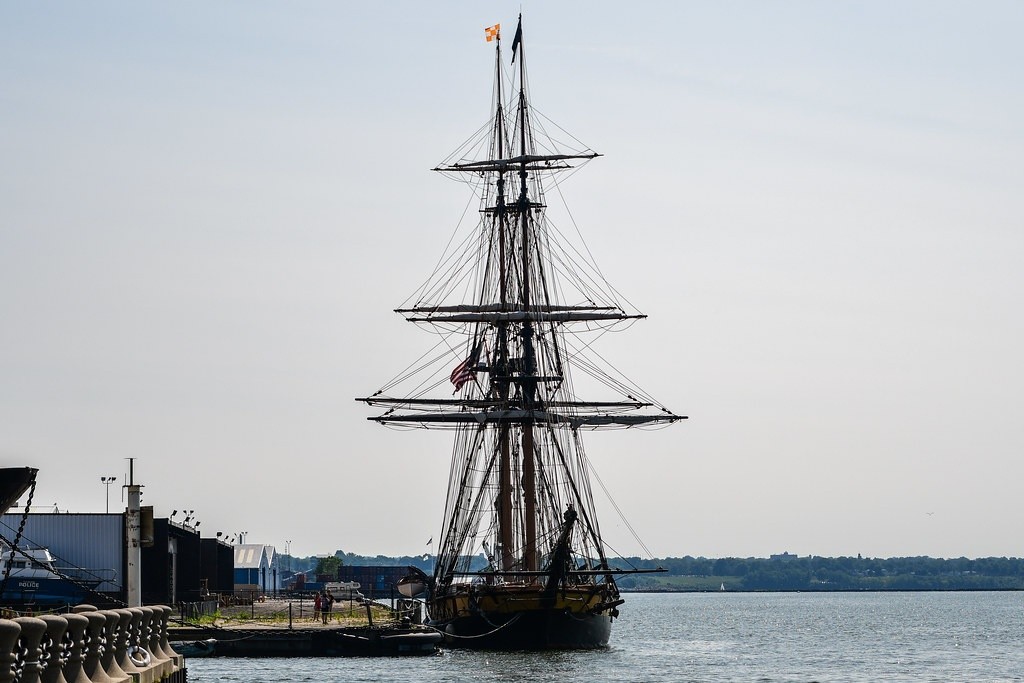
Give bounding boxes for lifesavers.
[126,644,152,669]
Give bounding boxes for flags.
[512,14,523,65]
[485,24,501,42]
[452,350,479,394]
[427,538,432,546]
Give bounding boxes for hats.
[325,588,331,592]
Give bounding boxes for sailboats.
[353,1,690,652]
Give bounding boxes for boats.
[0,543,117,617]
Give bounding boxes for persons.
[314,588,334,625]
